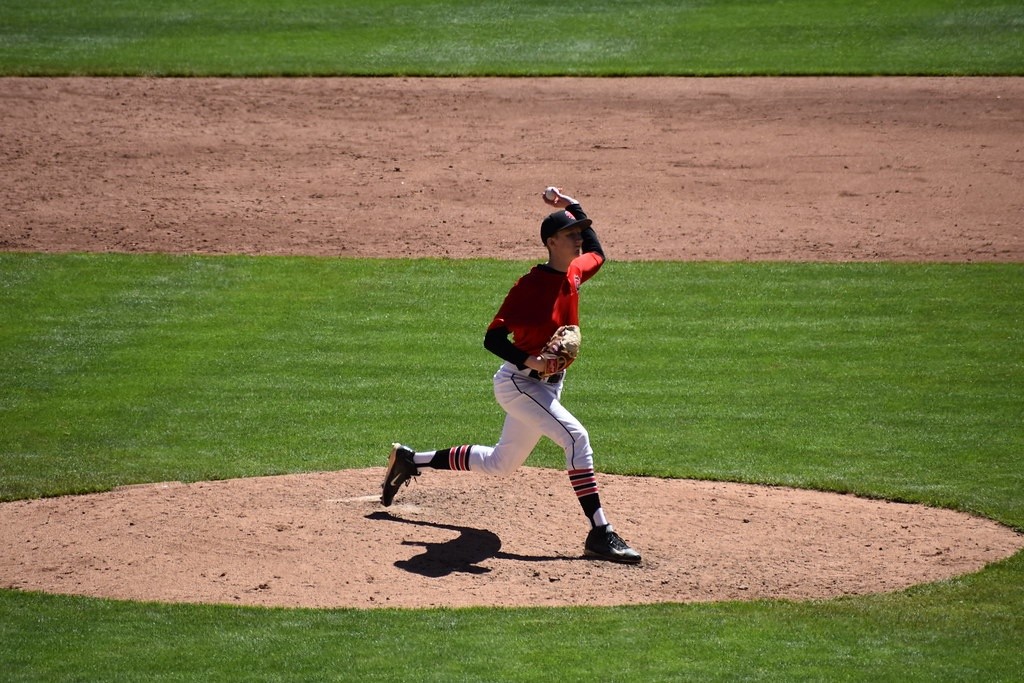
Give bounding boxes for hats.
[540,210,593,245]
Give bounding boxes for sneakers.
[380,442,422,507]
[583,528,641,563]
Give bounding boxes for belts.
[530,370,562,384]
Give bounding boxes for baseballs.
[544,186,559,202]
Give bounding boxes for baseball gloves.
[537,324,581,379]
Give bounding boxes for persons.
[381,185,642,563]
